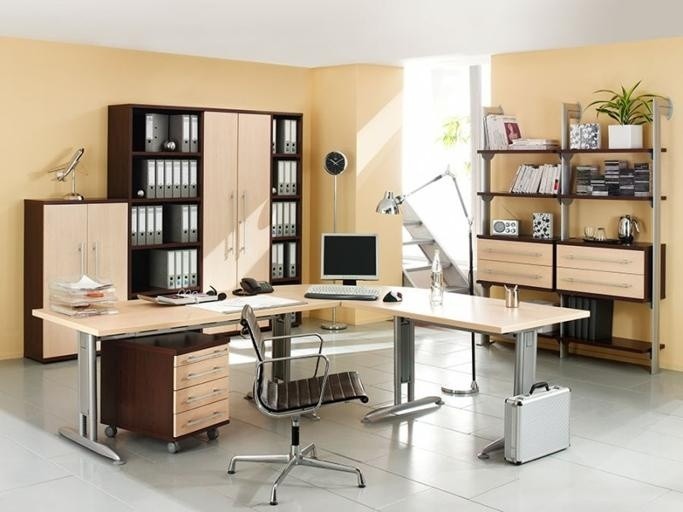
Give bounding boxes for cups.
[584,226,594,240]
[505,288,520,308]
[595,227,607,241]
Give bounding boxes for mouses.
[384,290,402,302]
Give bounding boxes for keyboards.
[303,285,383,300]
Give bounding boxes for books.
[483,105,560,152]
[136,287,218,305]
[49,277,119,318]
[507,163,560,195]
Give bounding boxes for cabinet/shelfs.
[200,106,273,293]
[106,100,204,300]
[98,332,234,456]
[271,110,303,289]
[472,91,671,377]
[21,195,130,366]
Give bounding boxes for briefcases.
[503,381,572,464]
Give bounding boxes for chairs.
[223,302,377,507]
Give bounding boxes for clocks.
[320,149,347,332]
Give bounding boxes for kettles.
[617,214,640,243]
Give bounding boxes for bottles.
[430,249,444,307]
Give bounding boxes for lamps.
[375,165,473,292]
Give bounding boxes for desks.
[29,280,593,472]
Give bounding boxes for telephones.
[240,278,274,294]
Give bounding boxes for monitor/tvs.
[320,232,382,285]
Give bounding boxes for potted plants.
[581,79,670,149]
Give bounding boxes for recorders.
[491,218,520,238]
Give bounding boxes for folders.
[163,204,198,243]
[271,201,296,237]
[271,119,296,154]
[270,242,296,279]
[148,249,198,290]
[134,159,197,198]
[145,113,199,153]
[273,160,297,196]
[130,205,163,246]
